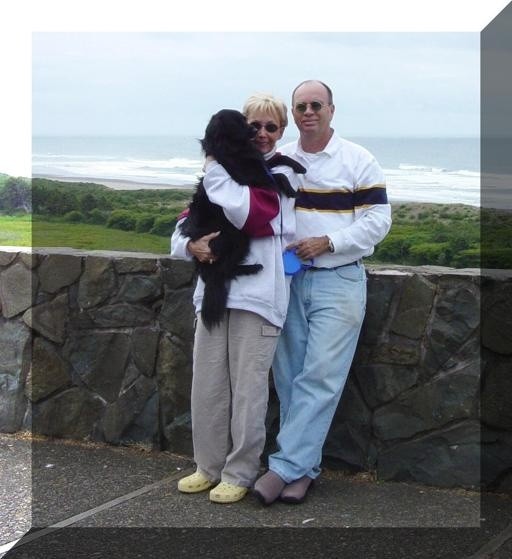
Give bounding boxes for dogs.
[175,107,307,336]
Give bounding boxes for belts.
[308,261,357,272]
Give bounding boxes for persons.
[252,80,393,508]
[170,95,308,504]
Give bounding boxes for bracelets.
[328,239,334,252]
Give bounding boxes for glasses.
[294,100,331,113]
[250,121,283,132]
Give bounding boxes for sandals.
[209,480,248,504]
[177,471,213,493]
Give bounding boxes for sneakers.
[253,469,315,505]
